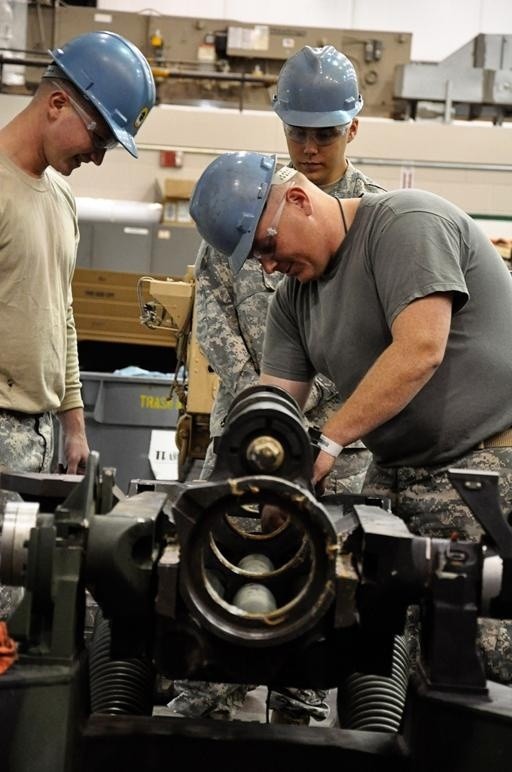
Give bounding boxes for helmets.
[273,46,363,128]
[189,151,278,275]
[47,30,155,159]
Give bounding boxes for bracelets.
[315,432,343,460]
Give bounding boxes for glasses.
[244,182,296,273]
[51,77,119,149]
[284,122,353,146]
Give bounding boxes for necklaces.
[324,192,351,243]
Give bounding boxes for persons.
[168,45,385,720]
[0,29,157,667]
[188,154,511,685]
